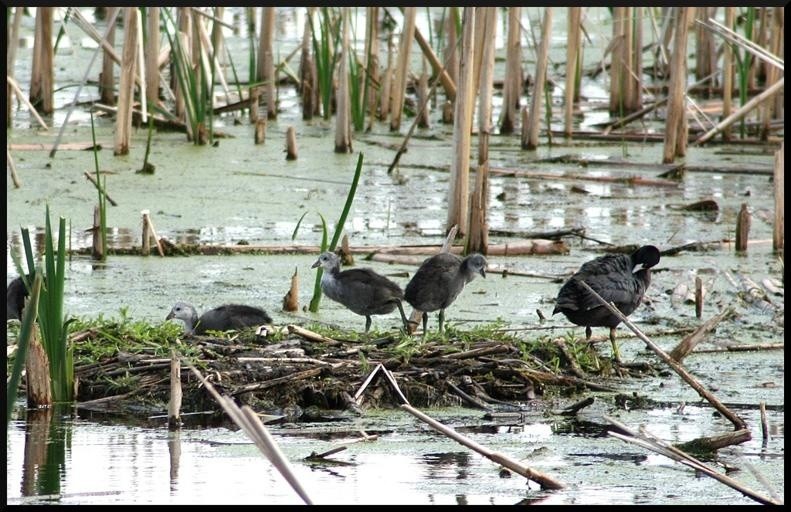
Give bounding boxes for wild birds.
[404,252,487,338]
[310,252,420,336]
[165,302,272,336]
[552,245,661,362]
